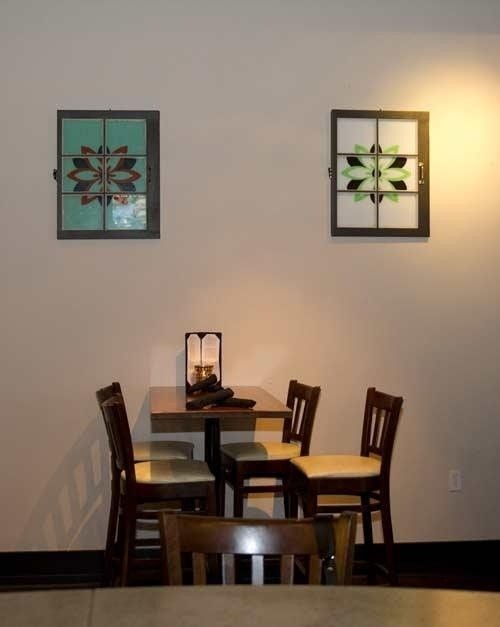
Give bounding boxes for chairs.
[288,385,402,580]
[222,379,321,520]
[95,380,214,584]
[157,504,358,585]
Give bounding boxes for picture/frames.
[330,110,429,235]
[56,111,160,239]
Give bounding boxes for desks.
[148,384,291,517]
